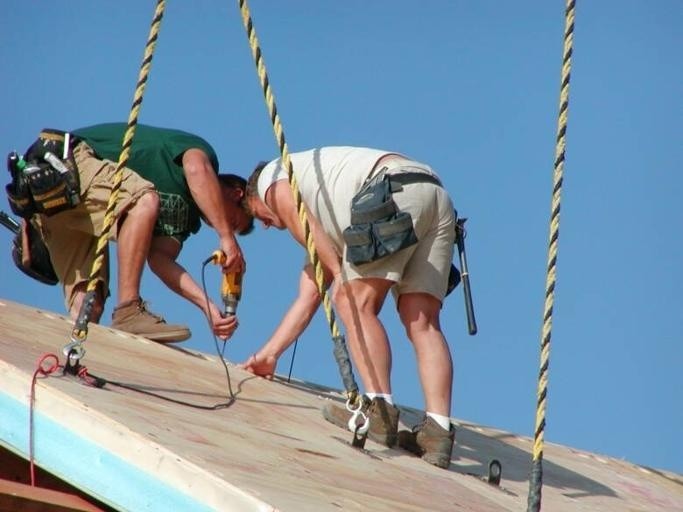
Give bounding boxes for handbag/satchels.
[5,128,81,285]
[342,166,419,265]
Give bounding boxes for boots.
[323,396,456,469]
[112,299,190,343]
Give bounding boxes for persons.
[28,122,252,342]
[234,146,456,469]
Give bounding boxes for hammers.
[453,218,477,334]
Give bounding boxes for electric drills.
[210,250,246,357]
